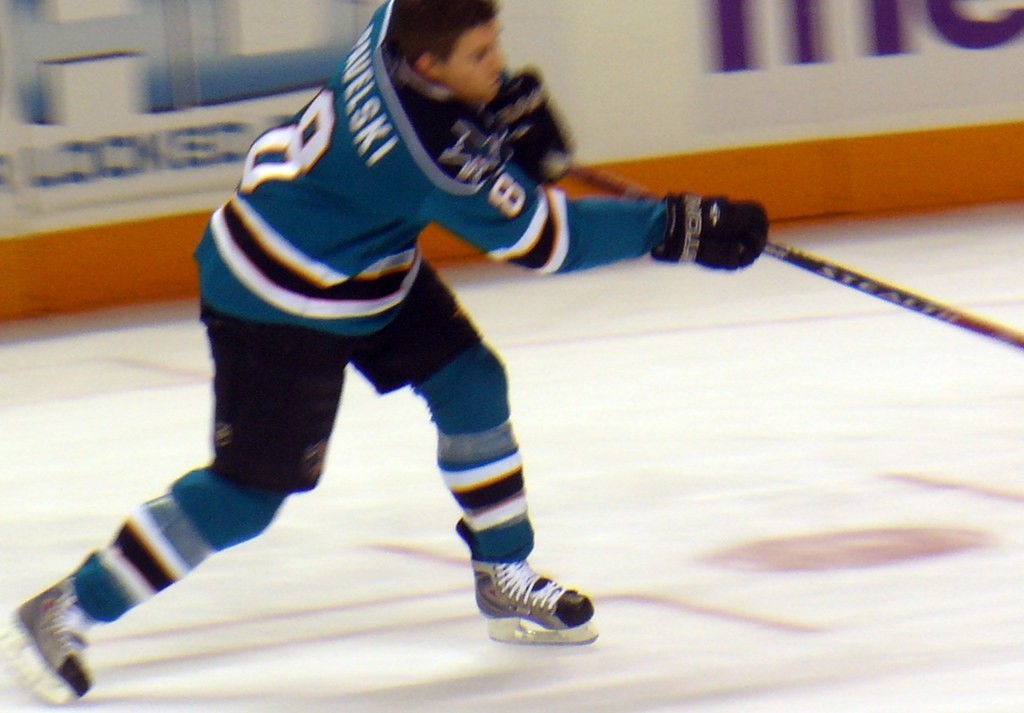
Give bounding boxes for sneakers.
[454,516,597,643]
[4,553,96,706]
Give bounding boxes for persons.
[17,0,771,696]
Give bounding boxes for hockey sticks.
[568,166,1024,351]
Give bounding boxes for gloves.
[480,65,572,187]
[651,193,769,271]
